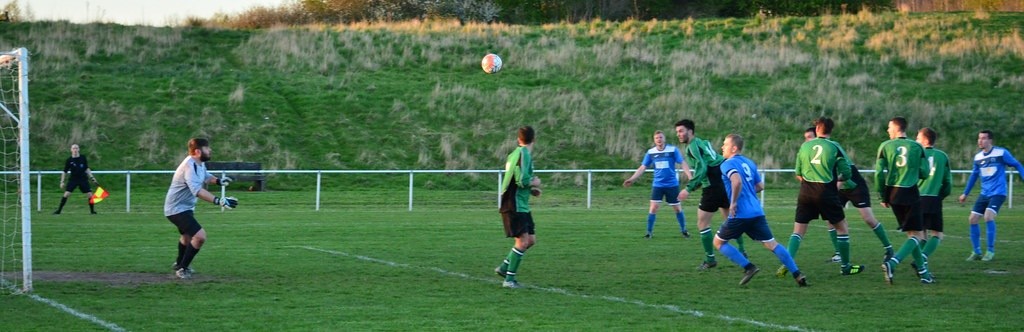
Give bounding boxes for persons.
[958,130,1024,262]
[909,124,953,278]
[494,126,543,289]
[801,127,894,264]
[163,138,239,279]
[776,116,866,275]
[712,135,809,287]
[673,118,749,270]
[52,144,102,215]
[623,131,693,238]
[873,117,938,284]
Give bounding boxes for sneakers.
[743,250,748,260]
[502,280,523,289]
[882,254,891,263]
[839,265,865,276]
[495,265,506,278]
[696,260,716,271]
[825,252,841,263]
[776,264,789,277]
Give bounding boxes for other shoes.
[981,250,994,262]
[683,231,690,237]
[966,252,982,262]
[910,253,927,271]
[739,266,759,285]
[642,234,651,238]
[53,210,60,215]
[920,276,938,284]
[881,262,893,285]
[796,274,807,287]
[176,268,190,280]
[91,211,96,213]
[171,262,195,273]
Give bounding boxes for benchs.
[205,161,266,192]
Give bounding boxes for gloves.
[213,197,238,213]
[216,177,233,187]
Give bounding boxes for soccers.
[481,53,503,74]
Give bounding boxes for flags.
[89,187,109,205]
